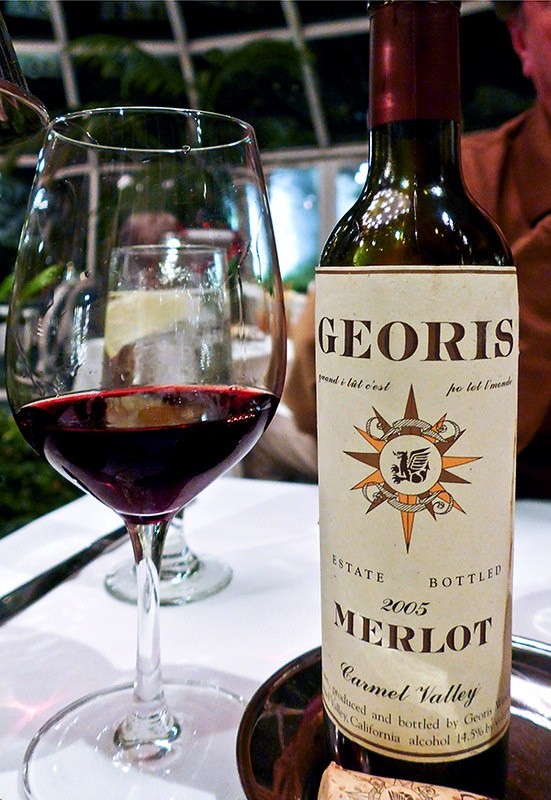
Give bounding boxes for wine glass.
[0,103,291,800]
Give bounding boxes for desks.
[0,478,551,800]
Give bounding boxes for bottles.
[311,0,522,800]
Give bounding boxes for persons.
[285,0,550,462]
